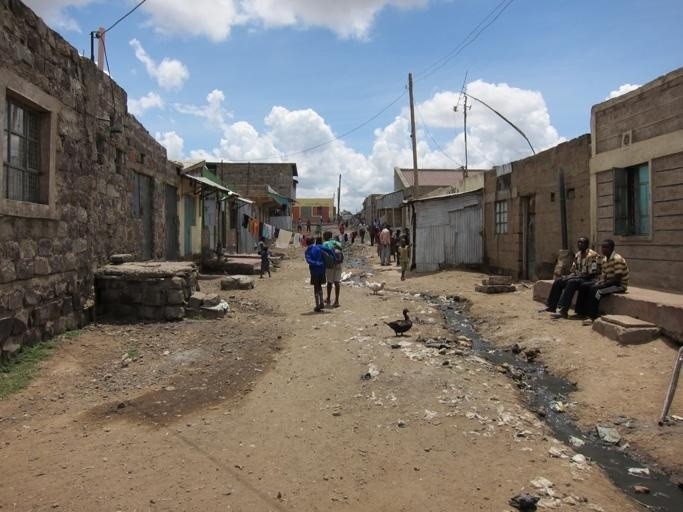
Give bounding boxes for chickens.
[365,280,386,295]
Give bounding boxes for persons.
[538,237,600,318]
[305,236,337,312]
[323,230,344,307]
[297,216,411,281]
[258,242,271,278]
[570,239,630,325]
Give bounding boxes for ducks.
[384,308,412,337]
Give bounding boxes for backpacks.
[329,240,343,264]
